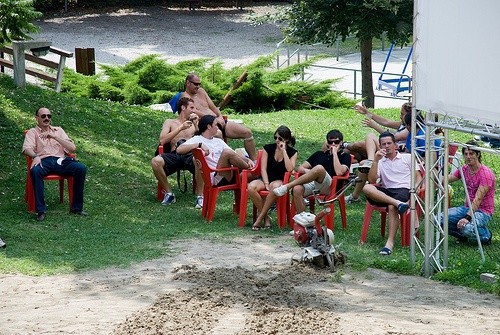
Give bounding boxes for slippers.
[251,217,273,230]
[397,202,409,213]
[378,248,394,255]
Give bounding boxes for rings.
[462,224,463,225]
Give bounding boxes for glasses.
[327,139,342,145]
[274,135,283,141]
[190,81,201,86]
[37,114,52,119]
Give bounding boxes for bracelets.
[33,154,39,159]
[464,214,472,222]
[264,182,269,185]
[198,142,202,148]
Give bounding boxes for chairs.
[157,144,195,201]
[24,130,77,211]
[289,153,354,229]
[360,164,427,247]
[239,150,295,228]
[190,148,247,222]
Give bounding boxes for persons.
[273,130,351,235]
[404,112,444,164]
[340,125,445,177]
[22,106,89,222]
[339,102,421,204]
[248,126,298,231]
[362,132,422,255]
[433,140,496,245]
[176,115,255,188]
[179,73,257,164]
[151,97,206,211]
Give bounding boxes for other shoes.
[69,208,89,216]
[357,163,372,172]
[344,193,362,203]
[195,196,204,209]
[273,185,288,197]
[37,212,46,221]
[160,192,177,206]
